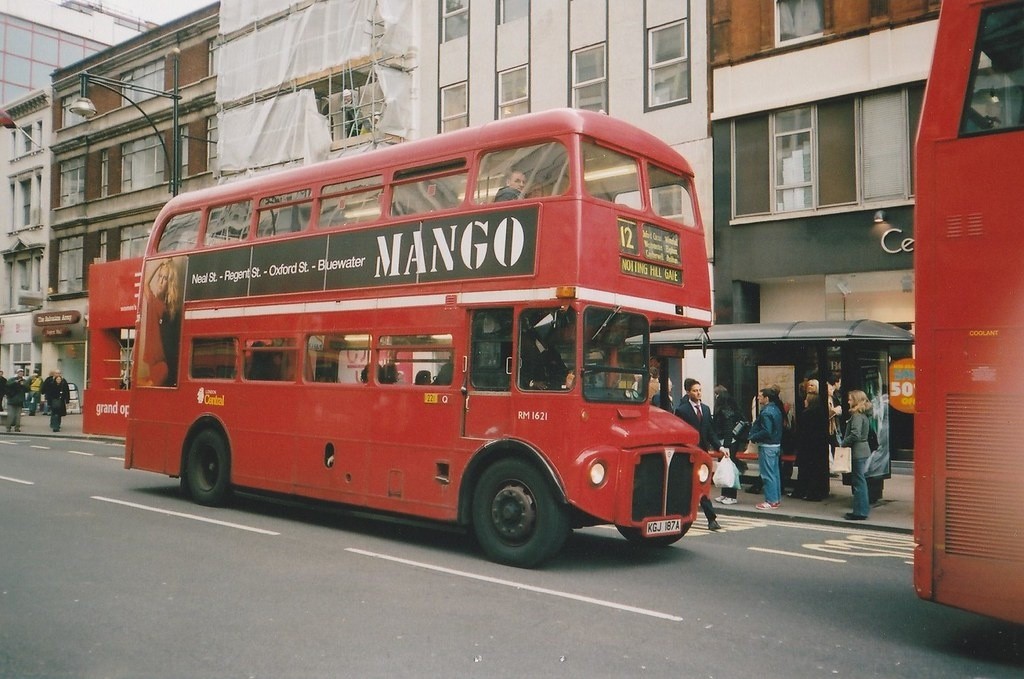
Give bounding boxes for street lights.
[66,47,183,197]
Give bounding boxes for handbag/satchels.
[730,414,750,443]
[832,447,852,473]
[713,455,742,490]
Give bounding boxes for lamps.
[874,209,887,223]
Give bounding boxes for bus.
[912,1,1024,627]
[125,106,719,569]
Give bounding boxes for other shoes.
[745,485,761,494]
[721,497,737,504]
[53,428,60,432]
[42,413,48,415]
[15,429,21,432]
[29,412,35,416]
[756,501,781,510]
[844,513,867,520]
[708,520,721,530]
[714,495,727,503]
[6,429,11,432]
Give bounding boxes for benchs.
[708,450,798,462]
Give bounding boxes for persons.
[6,369,26,432]
[494,170,543,202]
[711,385,748,504]
[40,370,70,431]
[675,381,730,530]
[746,384,788,494]
[0,371,8,411]
[520,315,568,390]
[134,257,181,386]
[747,388,782,509]
[361,361,399,384]
[415,370,432,384]
[632,367,674,411]
[25,369,44,415]
[679,377,711,419]
[842,390,872,521]
[795,373,842,478]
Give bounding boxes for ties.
[694,405,702,424]
